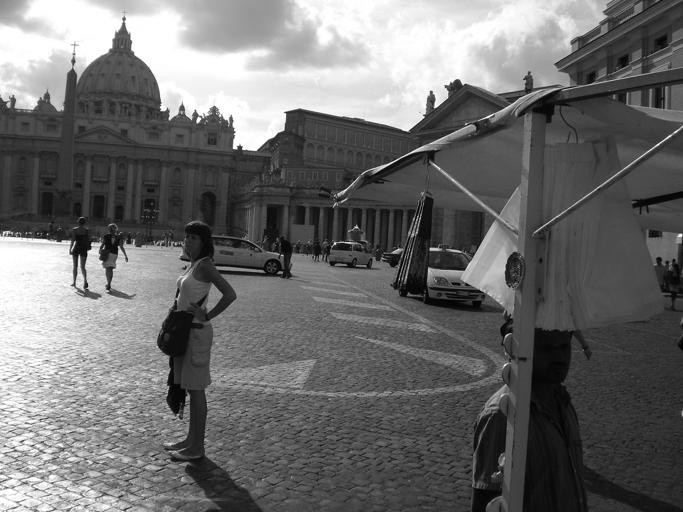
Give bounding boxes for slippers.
[163,443,178,449]
[170,450,201,460]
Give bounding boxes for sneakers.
[106,285,111,290]
[84,283,88,289]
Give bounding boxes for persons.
[162,222,237,461]
[426,90,436,117]
[523,71,533,94]
[261,235,334,262]
[69,216,91,288]
[470,311,588,512]
[9,95,16,108]
[229,115,233,127]
[278,236,293,279]
[654,257,680,310]
[99,223,128,290]
[191,109,199,123]
[47,221,54,241]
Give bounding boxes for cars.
[179,235,292,275]
[422,244,486,308]
[382,248,405,268]
[329,242,374,269]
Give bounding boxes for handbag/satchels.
[157,307,193,357]
[99,249,110,261]
